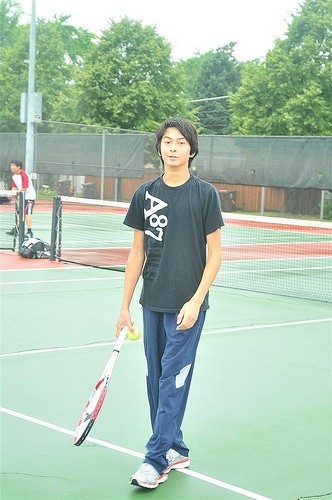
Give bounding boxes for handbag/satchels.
[20,236,49,258]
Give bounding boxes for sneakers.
[129,462,169,488]
[163,449,190,473]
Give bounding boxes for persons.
[115,118,225,490]
[6,160,36,239]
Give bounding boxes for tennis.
[126,325,141,341]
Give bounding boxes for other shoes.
[6,228,15,235]
[25,230,33,238]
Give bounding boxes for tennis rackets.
[0,181,16,202]
[72,316,134,447]
